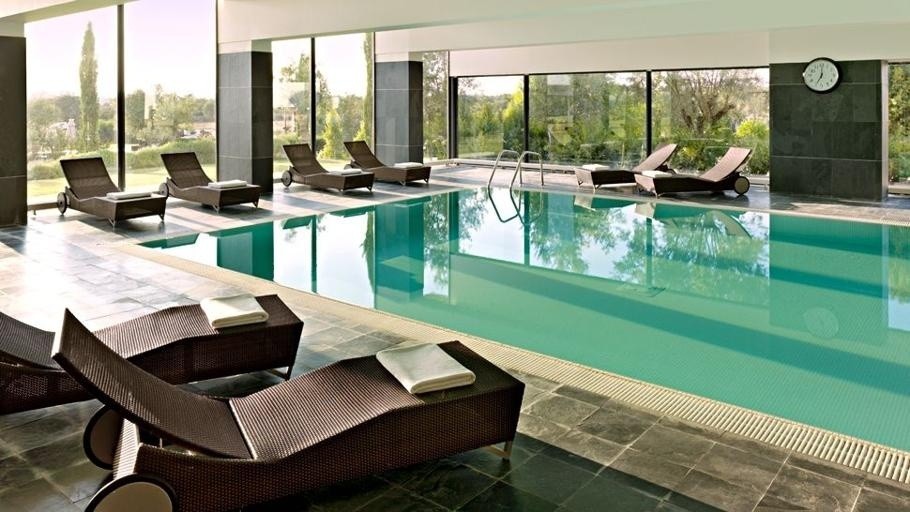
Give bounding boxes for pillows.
[640,170,673,178]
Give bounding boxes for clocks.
[802,307,839,338]
[803,56,842,93]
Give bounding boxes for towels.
[581,163,610,172]
[376,341,476,395]
[200,294,269,330]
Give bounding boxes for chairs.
[575,143,679,191]
[633,146,752,198]
[49,308,526,512]
[0,293,304,415]
